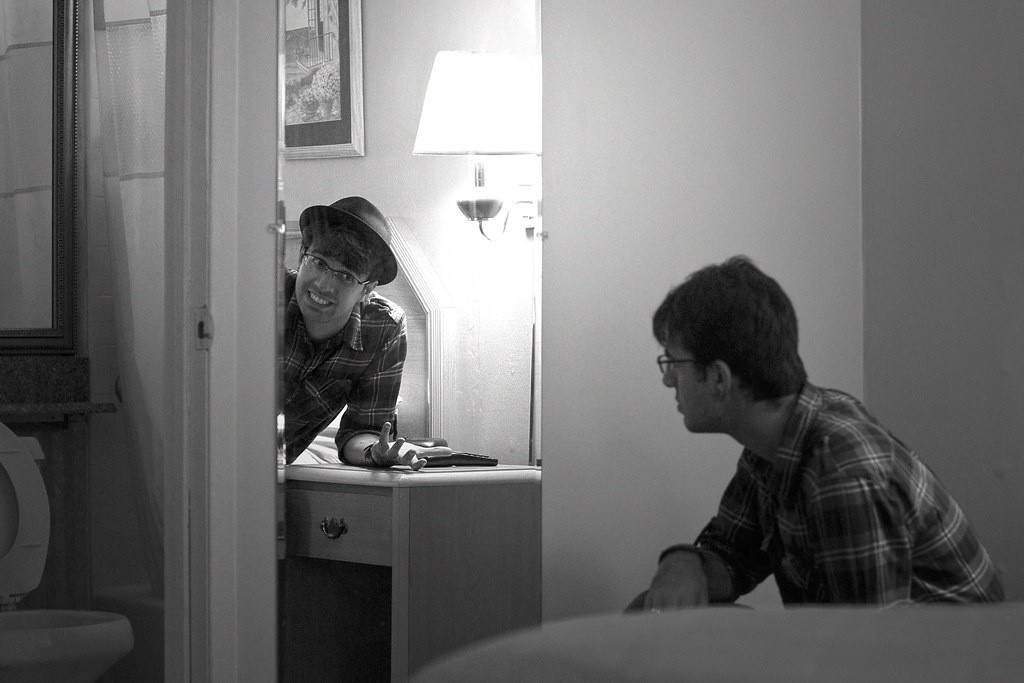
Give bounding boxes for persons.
[621,254,1007,621]
[278,194,428,485]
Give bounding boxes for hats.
[299,196,398,286]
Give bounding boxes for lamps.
[410,48,540,242]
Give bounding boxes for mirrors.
[272,0,540,683]
[0,0,79,359]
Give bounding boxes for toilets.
[0,421,135,683]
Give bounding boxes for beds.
[276,220,444,562]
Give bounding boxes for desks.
[287,464,543,683]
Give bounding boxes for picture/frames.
[285,0,366,160]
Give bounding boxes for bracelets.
[655,542,706,574]
[362,438,394,469]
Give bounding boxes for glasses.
[298,252,371,290]
[657,352,698,373]
